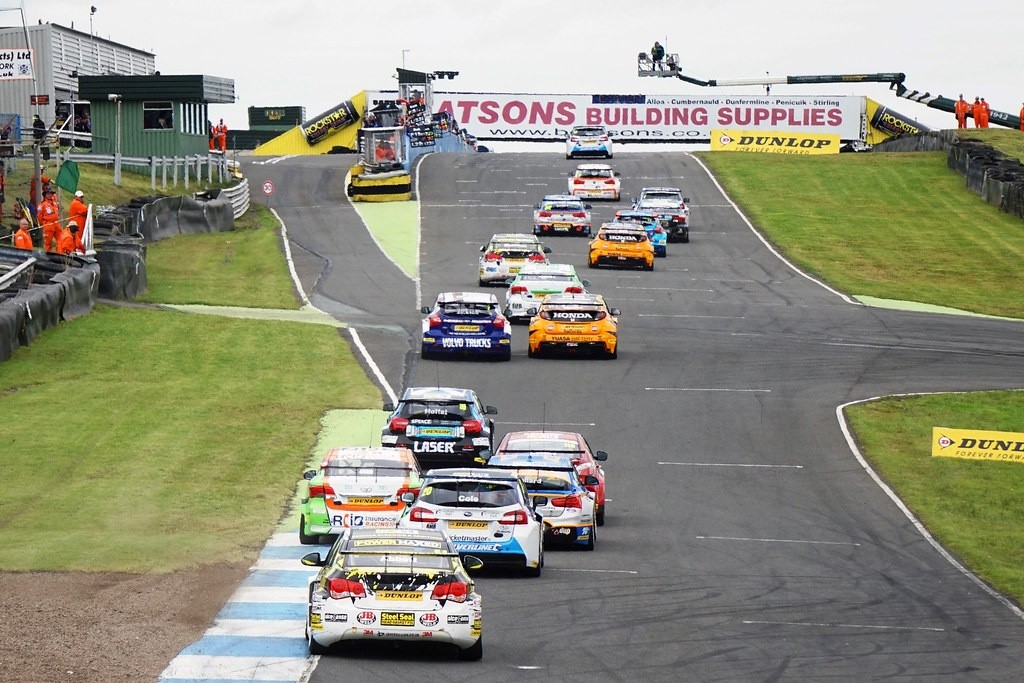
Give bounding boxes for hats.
[67,221,78,227]
[75,190,84,197]
[42,188,55,197]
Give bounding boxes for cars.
[419,292,511,361]
[532,195,593,237]
[301,527,484,659]
[480,233,552,288]
[502,262,592,323]
[564,125,614,159]
[567,164,622,201]
[487,430,607,551]
[299,446,426,546]
[587,186,691,271]
[527,292,622,359]
[380,387,497,468]
[401,467,548,578]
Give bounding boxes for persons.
[1020,103,1024,131]
[955,94,968,128]
[74,112,91,148]
[31,164,55,202]
[652,42,664,71]
[15,219,33,249]
[208,118,227,151]
[56,221,85,254]
[69,191,87,238]
[33,114,45,144]
[37,188,63,252]
[970,97,990,128]
[375,141,395,161]
[0,161,8,228]
[413,90,421,99]
[158,118,167,128]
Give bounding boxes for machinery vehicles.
[637,51,1021,129]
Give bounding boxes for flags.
[56,160,80,194]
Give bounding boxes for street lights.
[402,49,409,68]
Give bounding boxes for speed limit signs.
[263,182,273,194]
